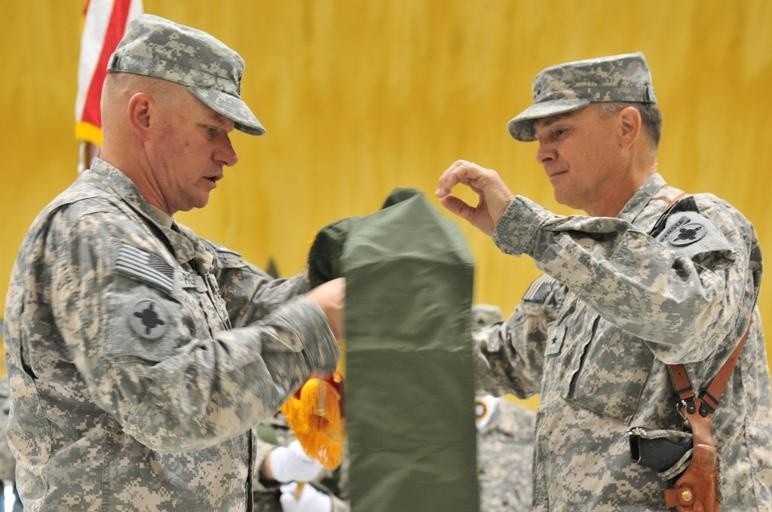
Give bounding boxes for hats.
[509,51,657,141]
[106,12,265,137]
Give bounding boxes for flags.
[75,1,142,148]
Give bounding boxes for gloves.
[277,482,332,511]
[269,439,323,482]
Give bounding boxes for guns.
[627,426,720,511]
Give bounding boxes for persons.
[437,54,770,511]
[4,12,343,512]
[251,411,349,512]
[469,306,538,512]
